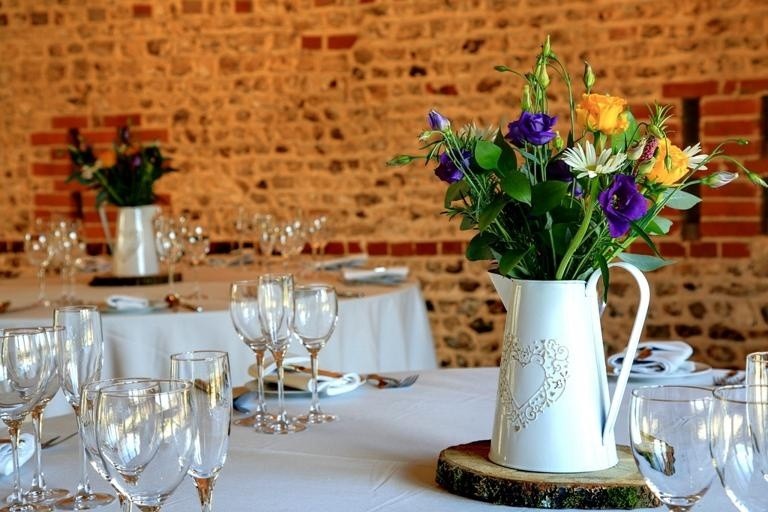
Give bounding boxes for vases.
[93,199,162,277]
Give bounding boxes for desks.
[2,366,767,512]
[1,263,435,421]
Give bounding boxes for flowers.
[67,126,179,204]
[383,27,768,281]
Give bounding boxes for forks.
[366,372,421,389]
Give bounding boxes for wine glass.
[4,326,71,507]
[97,378,196,512]
[170,352,234,512]
[287,284,339,427]
[79,378,165,511]
[256,272,307,436]
[53,305,114,512]
[1,328,53,509]
[228,280,278,430]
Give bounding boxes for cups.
[629,347,767,511]
